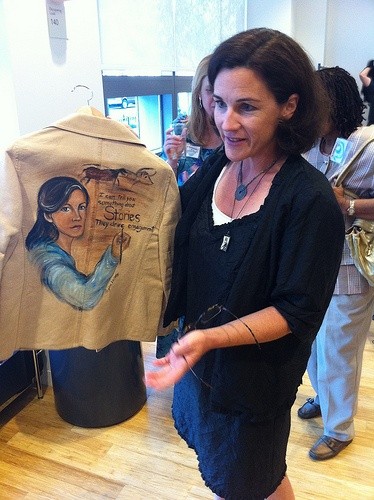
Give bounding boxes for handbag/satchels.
[345,218,374,286]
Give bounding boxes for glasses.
[178,303,261,391]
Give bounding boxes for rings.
[168,363,173,368]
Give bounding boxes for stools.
[49,340,148,428]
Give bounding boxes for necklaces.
[219,152,283,252]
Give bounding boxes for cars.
[108,96,135,109]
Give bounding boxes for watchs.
[347,199,356,215]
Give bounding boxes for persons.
[359,60,374,126]
[298,67,374,461]
[144,27,347,500]
[158,54,223,188]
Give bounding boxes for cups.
[169,123,187,160]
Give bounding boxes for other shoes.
[298,397,322,418]
[309,434,354,461]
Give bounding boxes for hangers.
[11,84,173,172]
